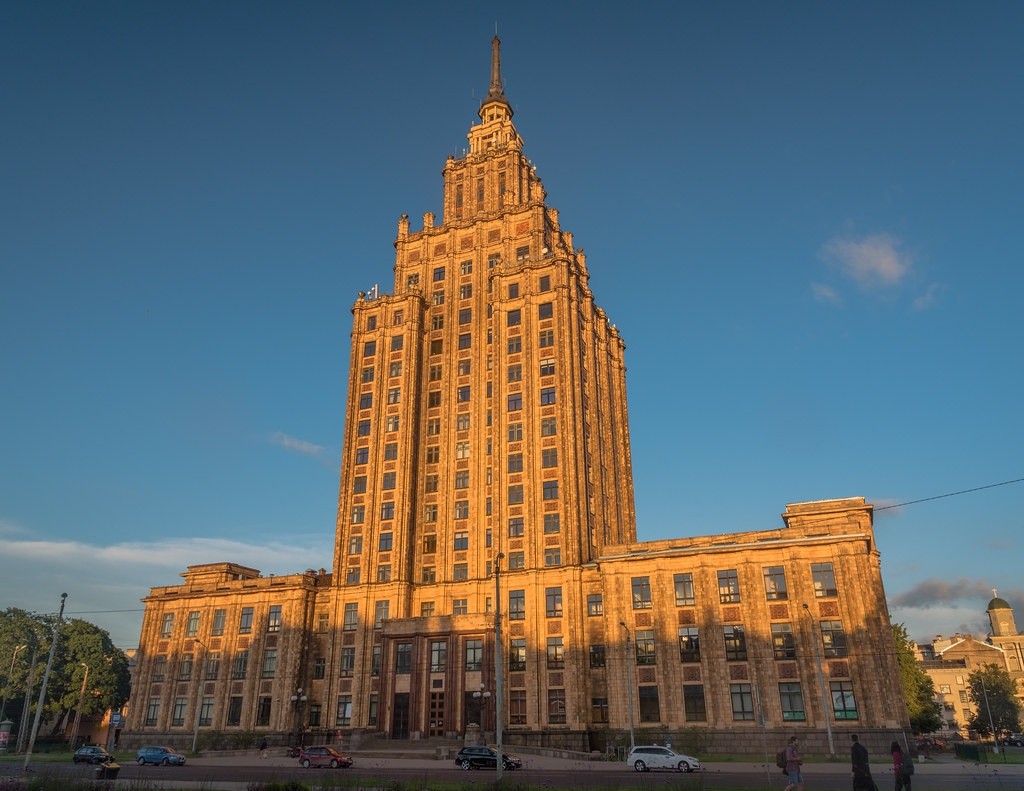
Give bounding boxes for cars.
[916,737,945,751]
[299,746,353,769]
[626,745,701,773]
[136,745,185,766]
[1001,735,1024,747]
[72,745,115,766]
[454,745,522,771]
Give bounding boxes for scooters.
[290,744,305,758]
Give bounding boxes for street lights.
[619,621,635,748]
[21,592,69,771]
[472,683,491,746]
[191,638,209,753]
[71,662,89,752]
[802,602,835,755]
[289,688,308,747]
[0,644,27,721]
[975,661,998,747]
[492,551,506,782]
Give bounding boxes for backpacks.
[895,750,914,776]
[777,747,793,768]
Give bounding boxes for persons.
[783,736,804,791]
[259,738,268,759]
[890,741,911,791]
[851,734,878,791]
[336,730,343,744]
